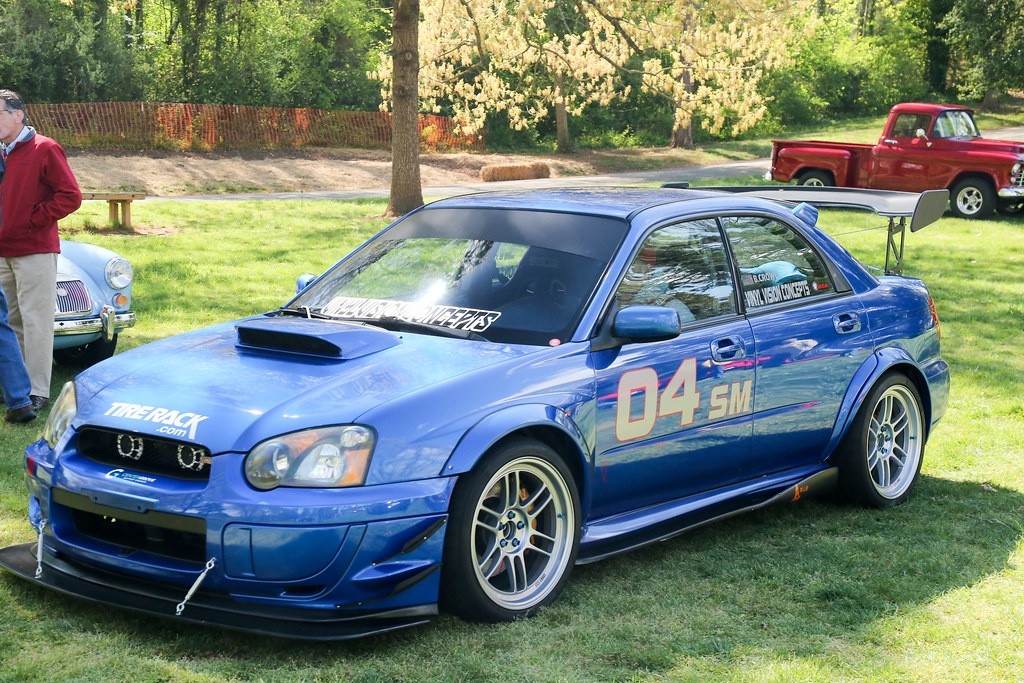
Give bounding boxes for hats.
[638,246,657,267]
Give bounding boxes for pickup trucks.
[770,101,1024,220]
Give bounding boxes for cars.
[54,238,136,368]
[0,180,950,642]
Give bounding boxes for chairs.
[654,245,714,314]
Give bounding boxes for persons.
[613,237,696,328]
[0,88,82,428]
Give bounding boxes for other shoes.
[5,404,36,422]
[30,395,49,409]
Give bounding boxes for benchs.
[81,192,146,231]
